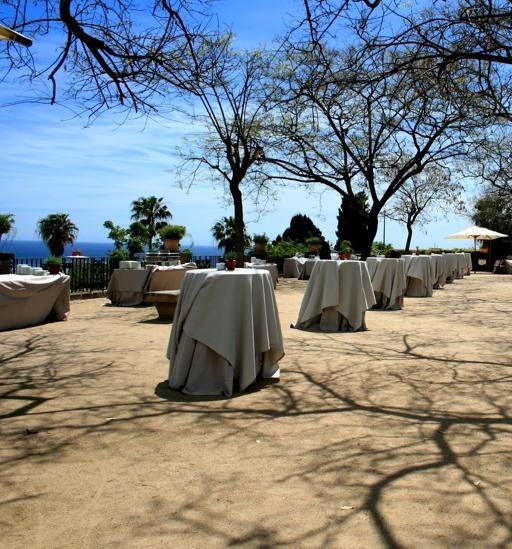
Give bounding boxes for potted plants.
[376,243,386,258]
[305,236,321,252]
[156,223,186,251]
[46,255,64,274]
[34,213,80,258]
[252,234,269,252]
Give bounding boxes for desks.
[402,255,433,297]
[109,269,149,307]
[147,258,198,320]
[0,270,65,330]
[244,255,322,287]
[291,260,377,332]
[365,257,406,310]
[167,268,286,394]
[428,253,470,288]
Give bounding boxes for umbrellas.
[445,225,510,274]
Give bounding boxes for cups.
[216,262,225,271]
